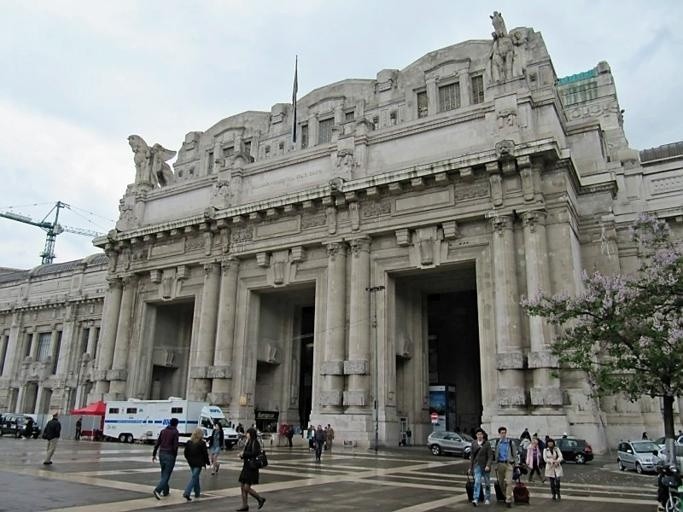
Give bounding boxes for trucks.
[102,397,239,449]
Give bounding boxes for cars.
[652,444,682,477]
[616,438,662,473]
[653,433,682,476]
[544,438,594,464]
[462,437,521,464]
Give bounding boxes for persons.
[150,418,180,501]
[183,428,209,500]
[466,428,494,507]
[76,418,82,440]
[286,420,334,463]
[641,432,648,440]
[561,432,568,438]
[41,411,61,465]
[238,427,266,512]
[25,417,34,438]
[495,426,517,506]
[542,439,564,500]
[209,422,223,474]
[658,464,683,512]
[406,427,412,447]
[520,428,554,484]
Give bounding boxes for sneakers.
[153,490,266,511]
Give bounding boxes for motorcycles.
[655,463,681,511]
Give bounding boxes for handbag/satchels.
[513,468,521,480]
[247,453,268,472]
[556,453,565,464]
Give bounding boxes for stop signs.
[429,413,438,421]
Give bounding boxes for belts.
[499,461,511,464]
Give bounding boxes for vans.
[0,413,41,439]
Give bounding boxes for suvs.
[427,431,475,460]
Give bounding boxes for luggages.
[466,472,484,501]
[494,469,506,503]
[514,477,529,505]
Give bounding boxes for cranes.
[0,201,116,265]
